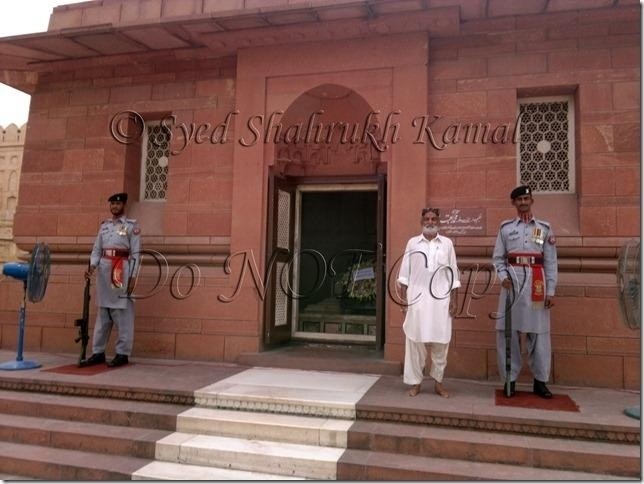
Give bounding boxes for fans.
[0,242,53,371]
[619,239,642,419]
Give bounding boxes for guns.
[505,291,511,398]
[74,278,91,364]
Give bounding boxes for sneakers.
[108,354,129,368]
[503,381,517,398]
[533,380,553,399]
[80,352,106,367]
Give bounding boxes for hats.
[108,192,128,201]
[511,185,532,199]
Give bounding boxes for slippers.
[433,386,449,398]
[408,385,421,397]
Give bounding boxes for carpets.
[495,389,580,414]
[41,362,135,376]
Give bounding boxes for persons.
[490,185,558,401]
[394,206,461,400]
[80,192,140,367]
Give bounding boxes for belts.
[508,257,544,265]
[102,249,129,257]
